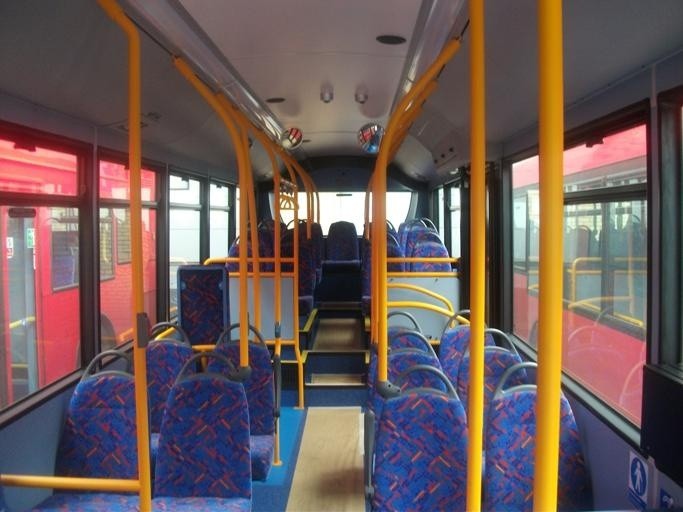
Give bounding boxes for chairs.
[24,209,601,511]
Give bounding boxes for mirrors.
[279,127,304,153]
[356,121,387,153]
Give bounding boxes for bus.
[0,137,191,409]
[510,123,650,437]
